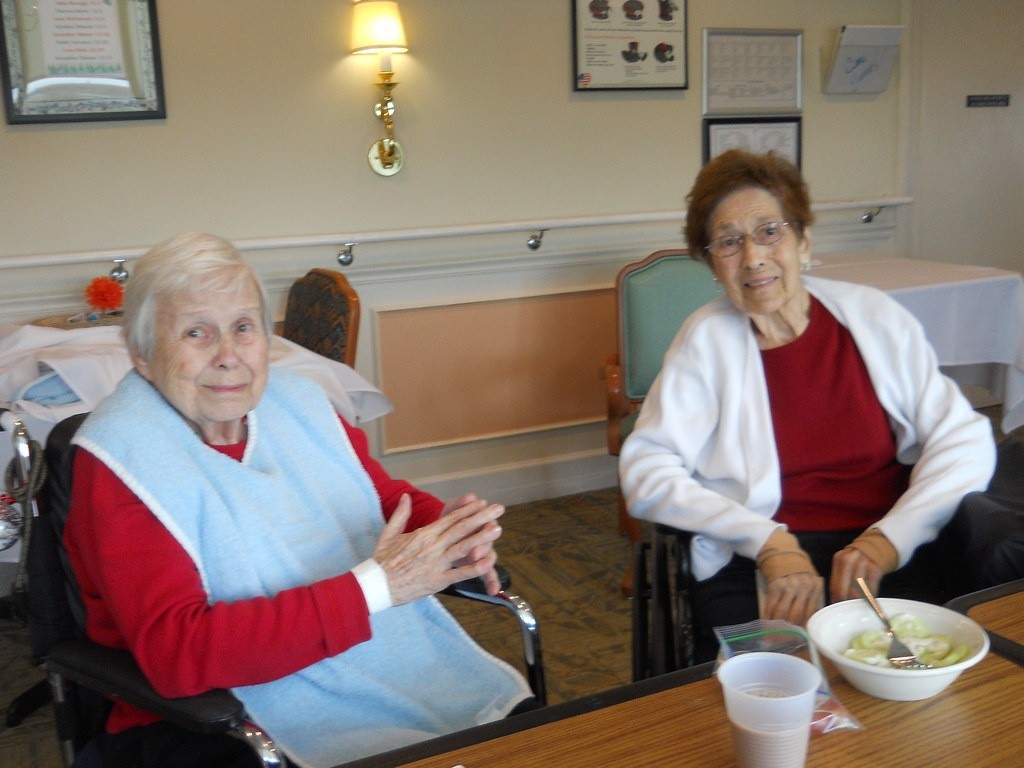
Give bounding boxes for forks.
[857,578,933,669]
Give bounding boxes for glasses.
[705,220,792,259]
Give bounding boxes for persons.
[618,144,996,666]
[60,231,545,767]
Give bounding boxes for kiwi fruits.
[842,612,969,671]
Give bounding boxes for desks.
[0,314,393,728]
[800,259,1024,435]
[330,577,1023,768]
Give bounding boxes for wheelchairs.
[12,412,547,768]
[632,428,1023,679]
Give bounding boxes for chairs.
[280,268,360,369]
[594,248,1024,592]
[5,412,547,768]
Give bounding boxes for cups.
[717,652,821,768]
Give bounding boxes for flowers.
[81,275,125,312]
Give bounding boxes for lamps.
[350,1,409,176]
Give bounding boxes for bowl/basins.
[806,597,990,701]
